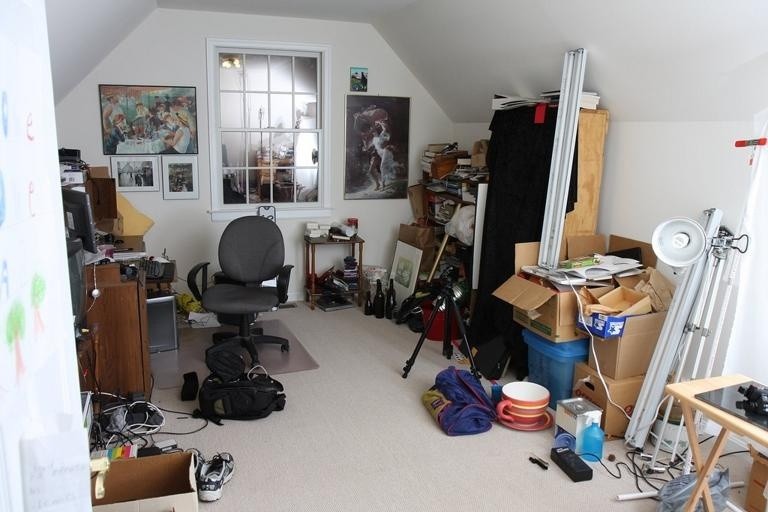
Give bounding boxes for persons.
[352,112,400,193]
[361,75,367,91]
[101,88,196,192]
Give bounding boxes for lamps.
[625,208,723,449]
[222,54,241,69]
[537,48,587,271]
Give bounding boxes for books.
[526,263,587,285]
[330,232,350,241]
[539,89,600,111]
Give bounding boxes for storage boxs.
[471,140,488,167]
[745,442,767,512]
[91,451,198,512]
[399,224,436,271]
[492,234,676,438]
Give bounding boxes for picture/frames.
[342,95,411,201]
[99,84,200,200]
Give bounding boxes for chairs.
[187,216,295,368]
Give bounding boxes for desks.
[664,374,768,512]
[304,236,363,310]
[85,235,151,402]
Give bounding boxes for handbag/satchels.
[459,318,515,380]
[421,366,497,436]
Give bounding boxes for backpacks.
[193,350,286,426]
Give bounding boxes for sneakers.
[185,448,234,501]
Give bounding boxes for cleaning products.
[582,410,604,462]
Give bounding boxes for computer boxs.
[146,295,178,354]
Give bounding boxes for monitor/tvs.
[91,177,118,220]
[61,185,100,254]
[67,236,89,325]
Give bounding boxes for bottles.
[364,278,397,319]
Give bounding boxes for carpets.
[151,319,320,389]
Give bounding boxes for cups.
[498,380,551,424]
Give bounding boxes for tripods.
[402,286,486,383]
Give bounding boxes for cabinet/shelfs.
[424,185,475,225]
[564,109,611,259]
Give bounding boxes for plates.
[496,410,553,432]
